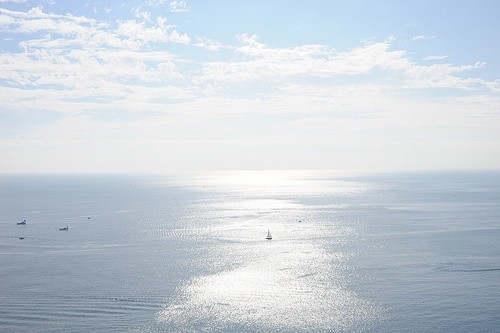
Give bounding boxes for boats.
[15,218,69,241]
[266,229,272,239]
